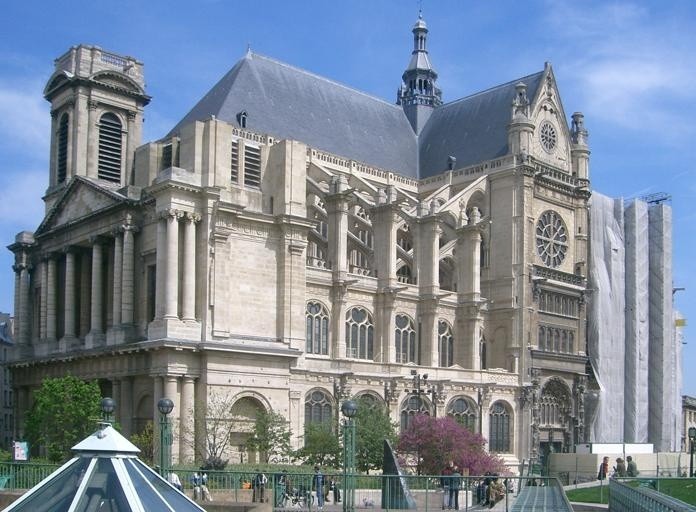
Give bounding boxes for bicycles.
[276,488,315,508]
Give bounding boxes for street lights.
[100,397,117,423]
[340,401,359,512]
[157,398,175,481]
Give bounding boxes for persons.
[598,457,609,480]
[278,465,343,510]
[440,465,462,511]
[166,469,184,494]
[250,471,268,503]
[613,458,627,477]
[476,470,515,509]
[626,456,637,477]
[189,468,214,502]
[528,477,545,487]
